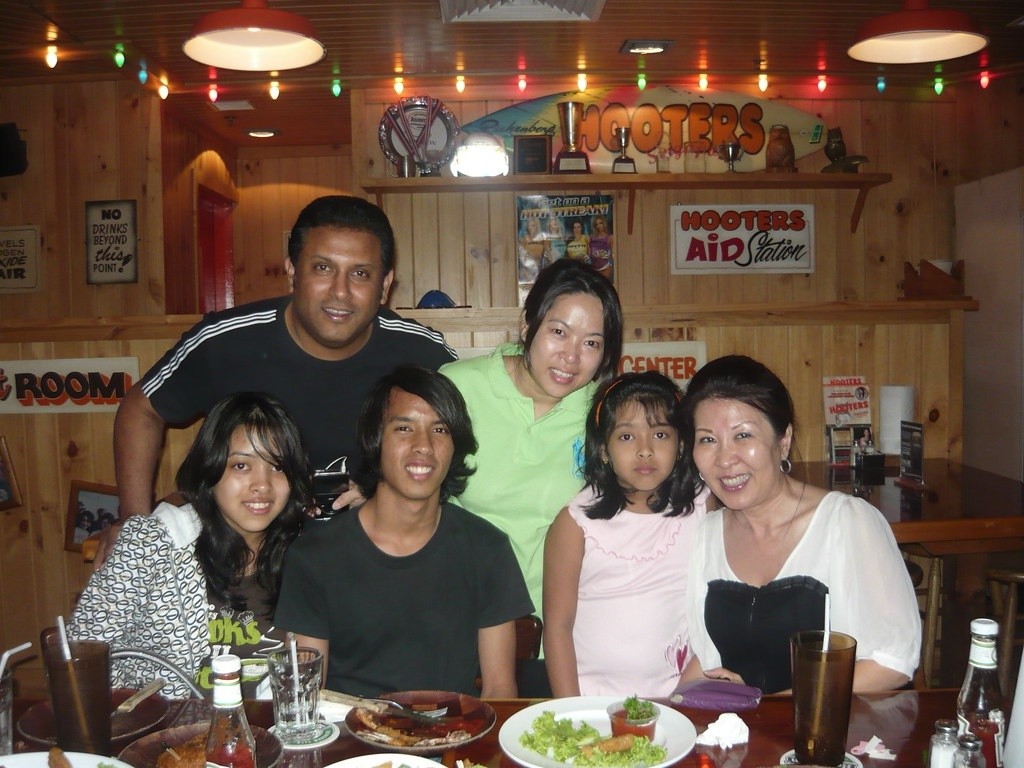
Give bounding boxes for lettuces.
[520,710,668,768]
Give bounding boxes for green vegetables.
[623,692,657,719]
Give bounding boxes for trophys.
[554,102,592,174]
[717,135,744,173]
[378,95,459,176]
[612,128,638,173]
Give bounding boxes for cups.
[789,627,857,768]
[43,639,112,757]
[266,646,323,733]
[606,699,660,746]
[0,665,12,759]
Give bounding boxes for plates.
[345,690,497,754]
[499,695,697,768]
[118,723,284,768]
[16,685,170,747]
[0,751,137,768]
[321,754,449,768]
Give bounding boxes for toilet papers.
[878,385,917,456]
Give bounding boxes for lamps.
[181,0,328,71]
[847,0,990,65]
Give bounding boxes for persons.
[89,195,459,574]
[65,393,315,699]
[517,216,615,274]
[669,355,922,700]
[860,429,871,446]
[286,362,518,702]
[438,256,623,700]
[74,511,114,545]
[543,370,718,698]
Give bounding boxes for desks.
[785,454,1024,688]
[9,690,1004,768]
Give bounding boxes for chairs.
[901,541,1024,705]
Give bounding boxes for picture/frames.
[63,480,122,555]
[0,436,23,512]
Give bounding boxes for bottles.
[927,719,960,768]
[204,655,258,768]
[956,618,1007,768]
[951,733,987,768]
